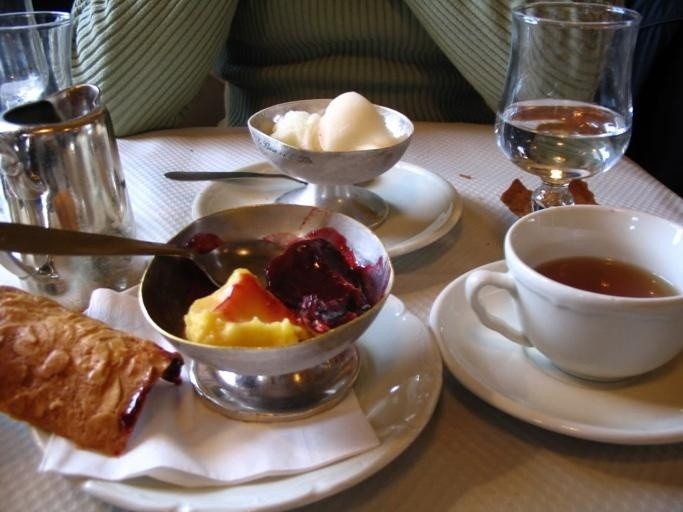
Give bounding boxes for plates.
[190,159,464,257]
[29,281,445,512]
[428,258,683,447]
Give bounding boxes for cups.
[0,79,149,314]
[493,0,644,209]
[462,201,683,384]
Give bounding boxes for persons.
[44,1,629,143]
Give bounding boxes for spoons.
[0,220,236,288]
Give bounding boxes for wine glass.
[136,202,397,425]
[246,96,417,231]
